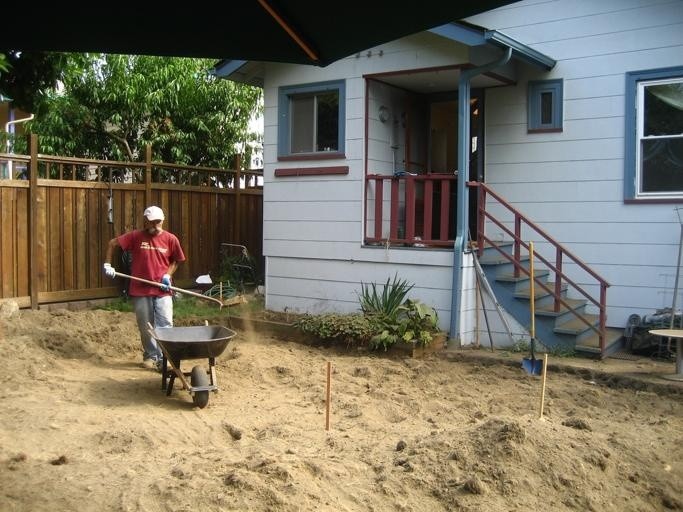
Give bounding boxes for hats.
[143,206,165,221]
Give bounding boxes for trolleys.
[148,322,238,411]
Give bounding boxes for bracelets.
[102,263,112,269]
[162,273,170,280]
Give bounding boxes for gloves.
[103,263,116,280]
[159,274,172,292]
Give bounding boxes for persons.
[104,205,185,373]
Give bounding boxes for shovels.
[520,241,544,379]
[537,353,548,423]
[111,270,243,308]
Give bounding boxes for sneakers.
[142,358,174,373]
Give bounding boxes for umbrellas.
[0,1,522,69]
